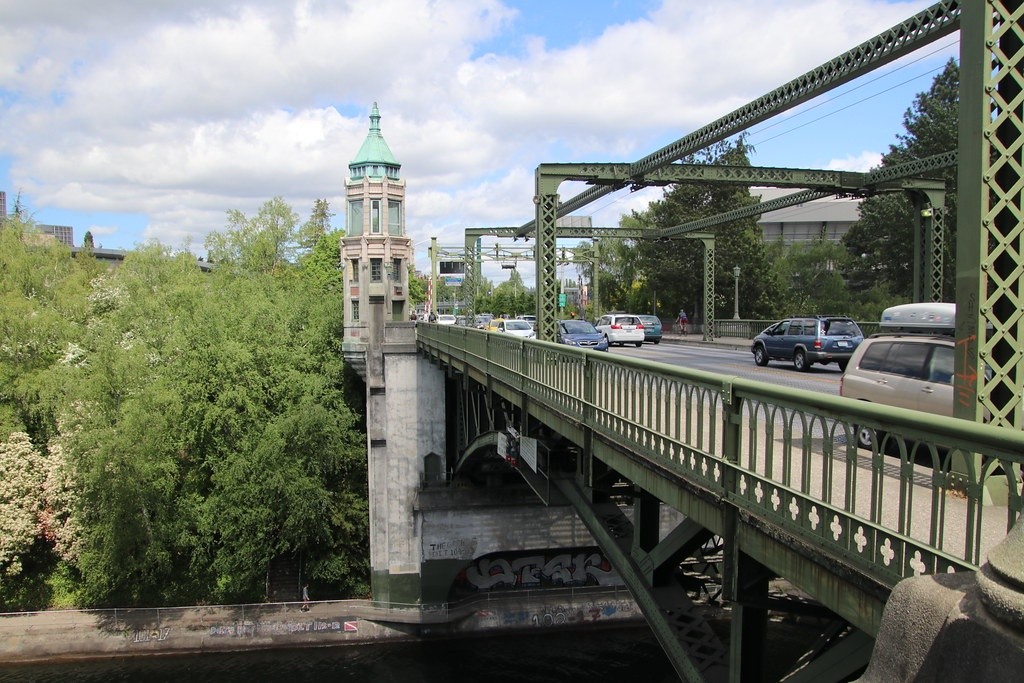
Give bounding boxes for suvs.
[751,314,863,372]
[838,302,1024,470]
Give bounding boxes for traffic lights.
[505,435,520,468]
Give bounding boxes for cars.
[497,319,536,349]
[472,316,493,329]
[484,318,504,335]
[479,314,494,319]
[437,315,456,325]
[556,320,608,352]
[622,315,663,344]
[455,316,472,327]
[594,314,645,347]
[417,314,428,321]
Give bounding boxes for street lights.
[732,265,741,318]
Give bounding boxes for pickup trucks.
[516,315,537,330]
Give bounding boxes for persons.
[428,312,436,323]
[571,311,574,320]
[300,584,312,613]
[676,309,688,331]
[411,312,417,321]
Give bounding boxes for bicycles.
[675,320,688,337]
